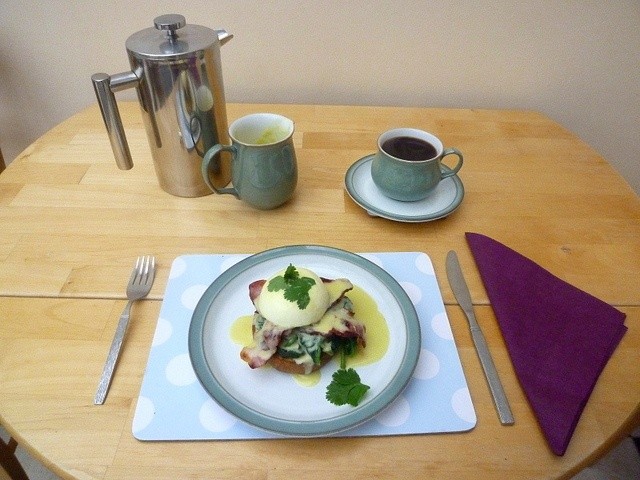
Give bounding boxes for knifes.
[445,251,517,427]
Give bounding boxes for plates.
[188,243,423,438]
[344,151,464,224]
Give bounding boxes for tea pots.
[90,14,235,199]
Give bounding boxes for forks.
[94,255,156,413]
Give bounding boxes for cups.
[201,114,300,213]
[371,127,463,203]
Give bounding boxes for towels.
[466,231,627,456]
